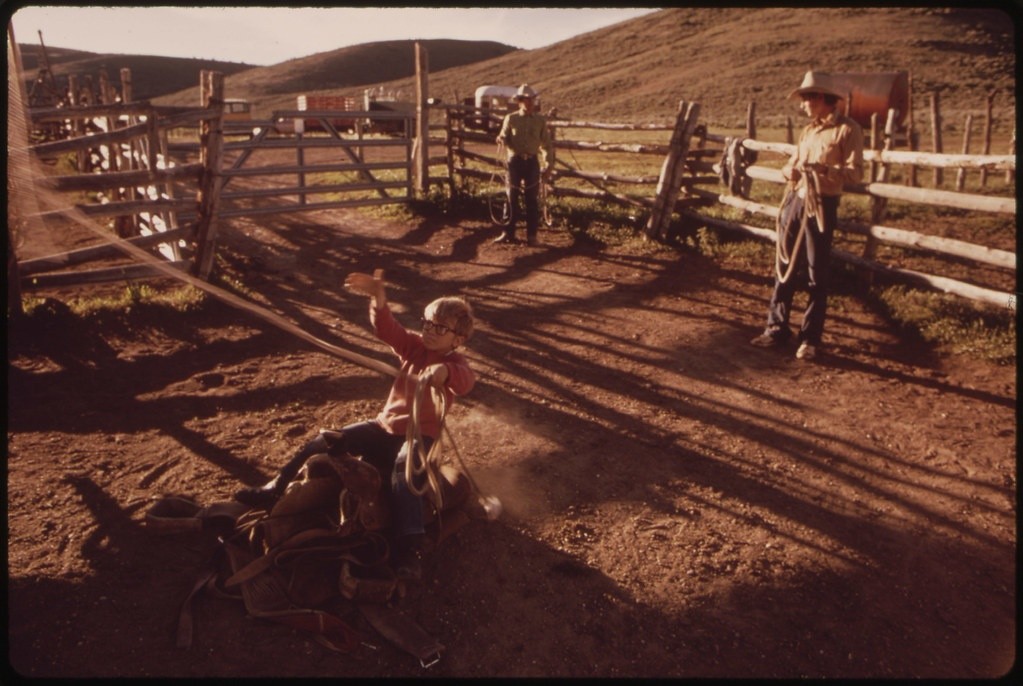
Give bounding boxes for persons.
[495,85,556,249]
[234,272,475,578]
[750,68,864,361]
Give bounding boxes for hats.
[787,70,844,102]
[512,84,539,103]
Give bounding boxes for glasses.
[420,317,460,337]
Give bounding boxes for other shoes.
[494,231,515,243]
[395,550,421,580]
[234,486,284,515]
[527,237,540,247]
[750,335,775,347]
[796,343,816,360]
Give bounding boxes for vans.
[221,98,254,121]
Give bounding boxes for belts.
[514,153,535,161]
